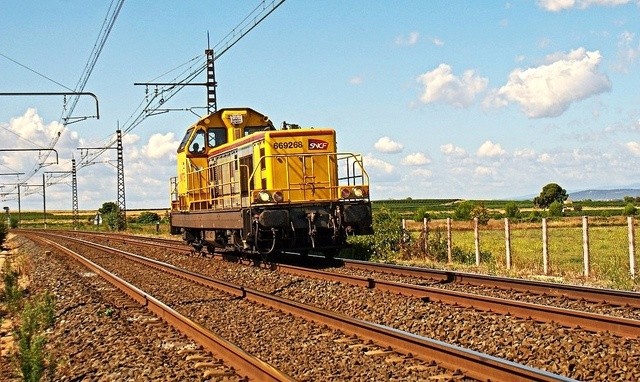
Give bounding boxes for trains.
[169,107,374,259]
